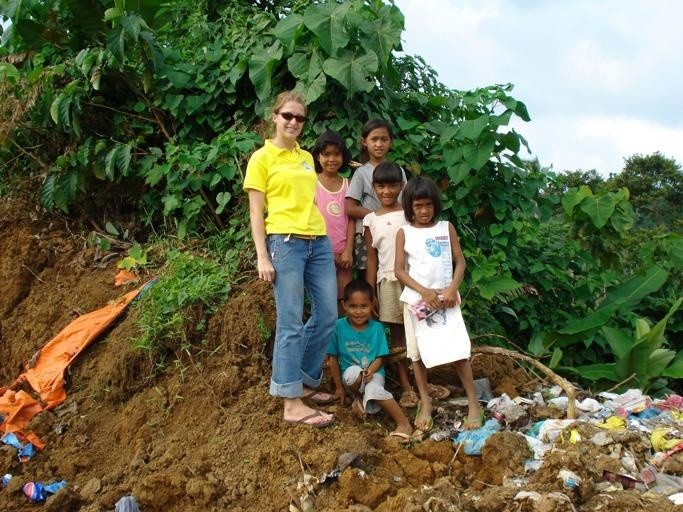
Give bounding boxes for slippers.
[284,409,336,427]
[414,397,432,432]
[425,382,450,399]
[399,386,419,408]
[388,431,412,443]
[464,407,485,427]
[302,389,335,403]
[351,398,367,416]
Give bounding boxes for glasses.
[277,111,307,123]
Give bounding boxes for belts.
[284,233,319,243]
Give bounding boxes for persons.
[361,161,449,409]
[323,279,412,446]
[313,128,357,320]
[237,90,339,429]
[391,174,485,433]
[343,116,407,282]
